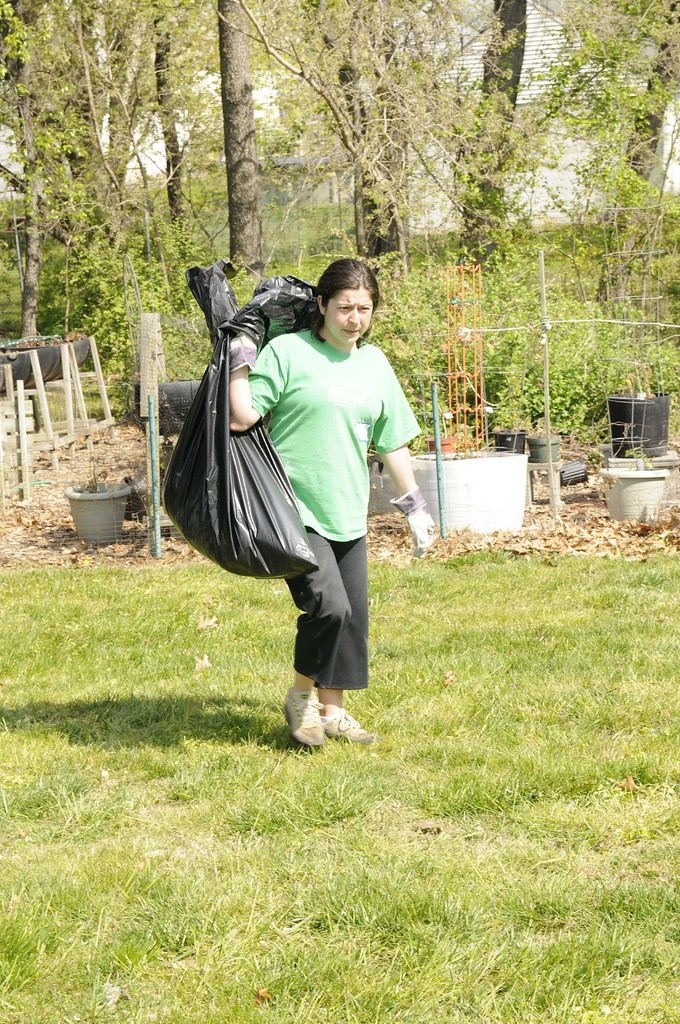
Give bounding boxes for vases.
[494,429,529,453]
[132,380,204,437]
[607,393,671,456]
[66,480,131,545]
[599,467,670,522]
[425,438,455,452]
[527,435,559,462]
[411,451,527,531]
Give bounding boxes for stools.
[527,458,563,506]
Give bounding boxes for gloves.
[390,486,438,557]
[226,325,257,374]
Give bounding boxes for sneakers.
[284,689,326,748]
[321,710,375,748]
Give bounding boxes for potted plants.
[560,451,606,487]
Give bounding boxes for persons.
[225,260,435,745]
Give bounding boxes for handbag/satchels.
[161,258,322,577]
